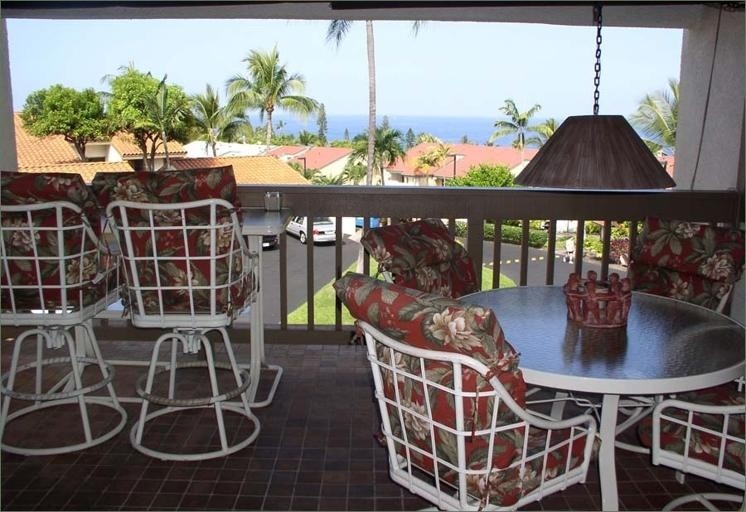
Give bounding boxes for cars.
[355,216,380,229]
[262,235,277,250]
[286,214,336,244]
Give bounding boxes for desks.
[97,203,294,408]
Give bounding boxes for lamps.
[512,1,677,190]
